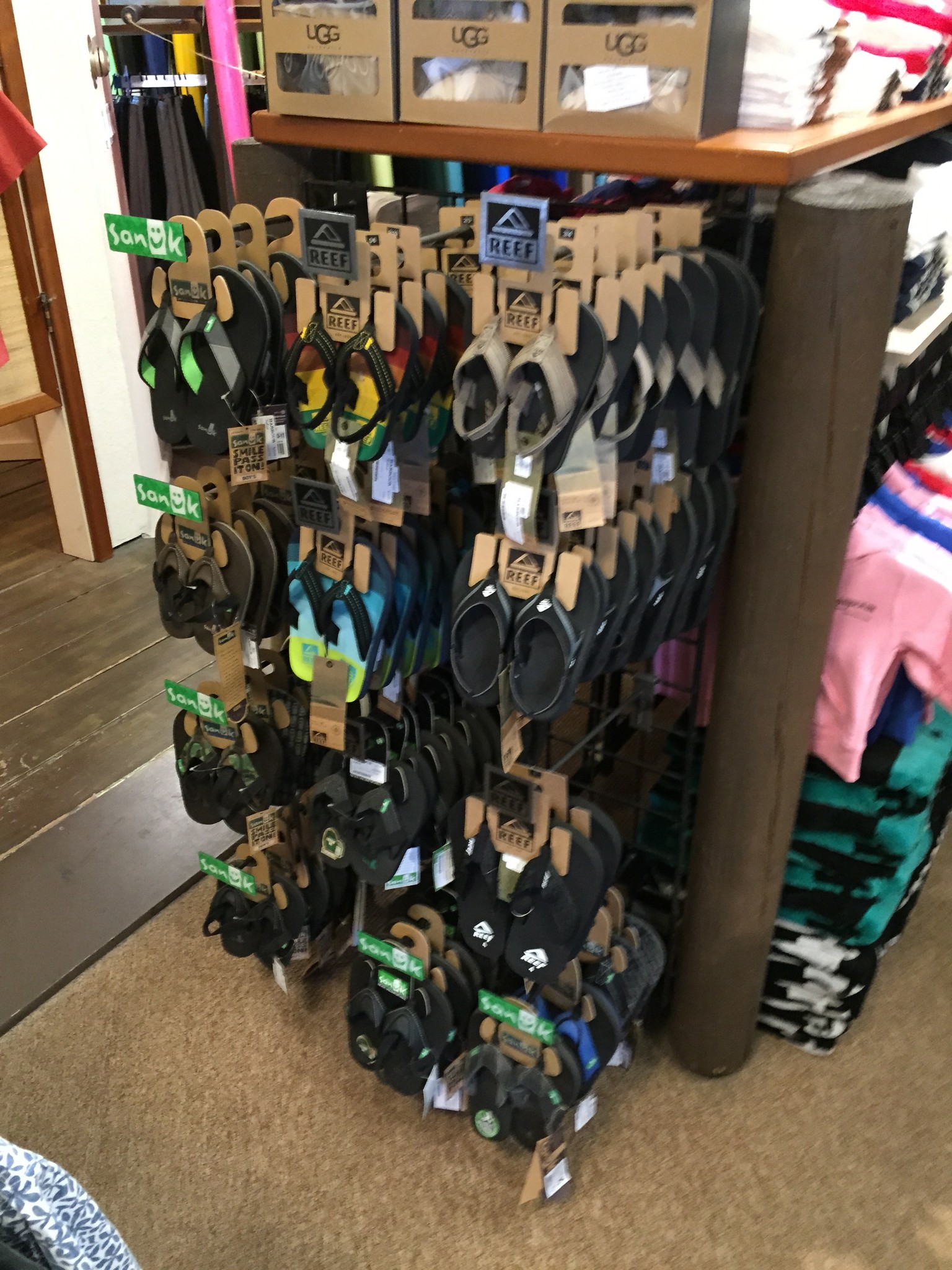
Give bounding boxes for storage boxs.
[261,0,750,141]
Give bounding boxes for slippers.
[170,675,668,1153]
[139,243,764,721]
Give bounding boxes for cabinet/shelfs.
[231,91,952,1076]
